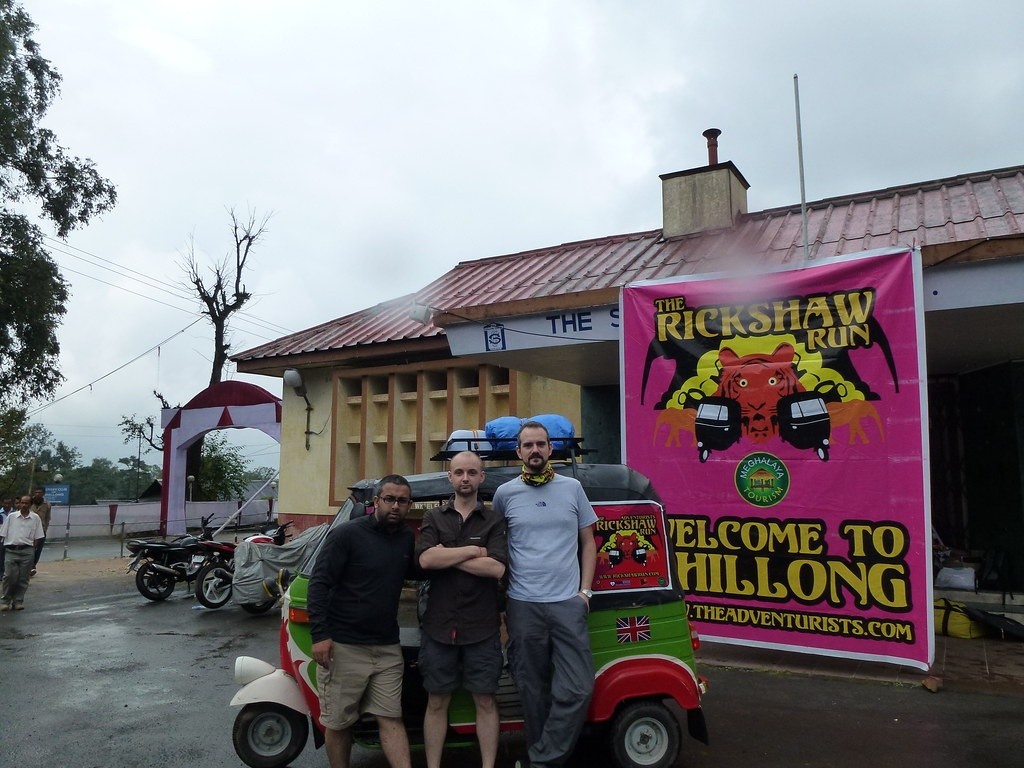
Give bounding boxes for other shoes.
[0,604,9,610]
[515,759,548,768]
[12,603,24,610]
[30,569,36,576]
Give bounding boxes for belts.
[6,545,28,550]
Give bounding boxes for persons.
[490,421,598,768]
[418,450,509,768]
[306,474,428,768]
[31,488,51,565]
[0,495,46,610]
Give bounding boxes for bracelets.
[480,547,482,556]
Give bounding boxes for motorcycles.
[126,513,331,614]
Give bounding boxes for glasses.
[377,495,410,507]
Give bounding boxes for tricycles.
[228,437,710,768]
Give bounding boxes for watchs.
[580,589,593,598]
